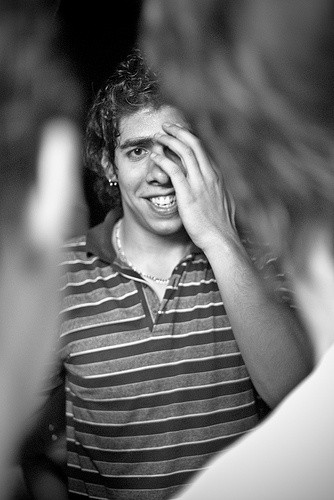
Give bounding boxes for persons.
[0,1,80,500]
[36,45,314,500]
[141,0,334,500]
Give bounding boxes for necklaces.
[115,218,172,283]
